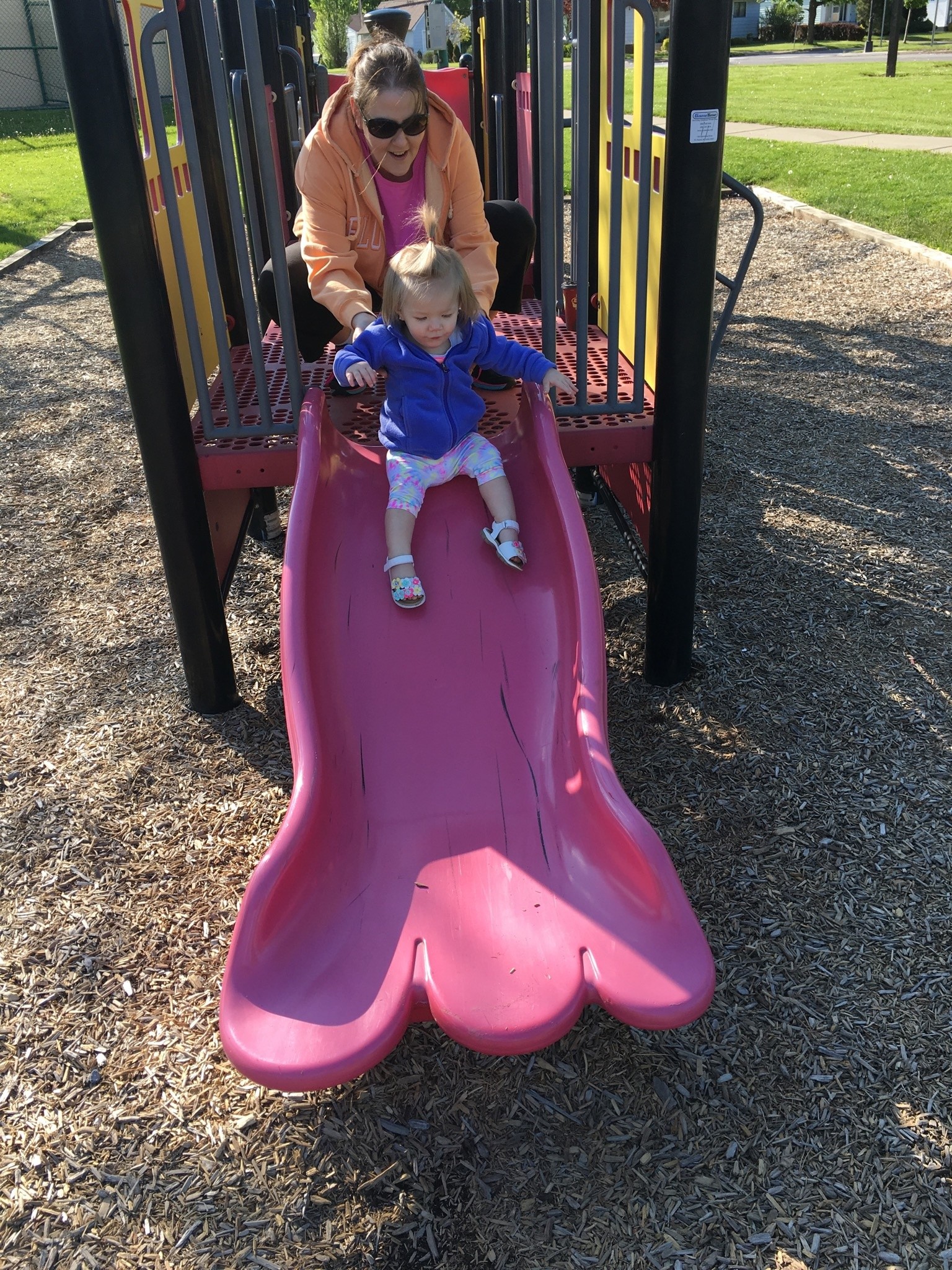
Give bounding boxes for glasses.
[357,100,429,139]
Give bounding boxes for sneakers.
[471,364,517,391]
[323,343,367,395]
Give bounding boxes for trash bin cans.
[437,49,448,69]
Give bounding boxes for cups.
[561,281,590,331]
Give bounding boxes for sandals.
[384,554,426,609]
[481,520,527,572]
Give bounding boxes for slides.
[216,379,719,1098]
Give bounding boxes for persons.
[257,25,538,396]
[333,204,579,608]
